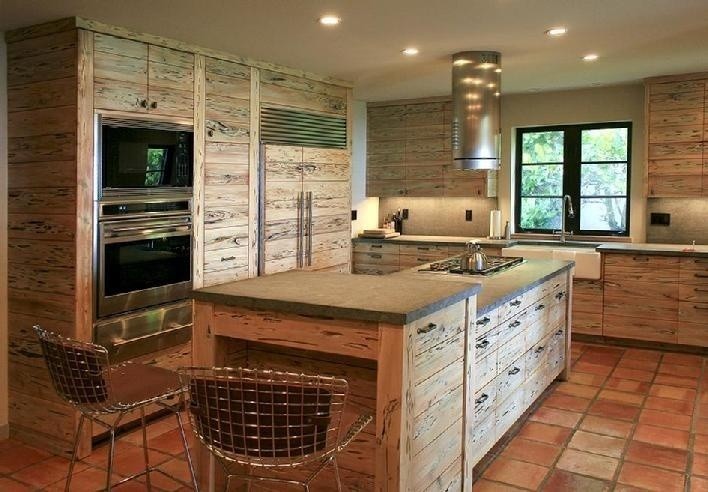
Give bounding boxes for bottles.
[382,207,402,235]
[505,220,511,240]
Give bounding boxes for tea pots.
[460,239,488,270]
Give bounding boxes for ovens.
[93,113,195,318]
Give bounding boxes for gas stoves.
[418,252,523,278]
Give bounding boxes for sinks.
[502,245,601,280]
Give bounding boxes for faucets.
[562,195,575,232]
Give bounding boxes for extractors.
[450,50,503,173]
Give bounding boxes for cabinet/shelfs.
[495,291,525,444]
[368,111,443,196]
[404,298,465,491]
[471,309,496,469]
[203,55,251,140]
[261,143,353,276]
[205,140,250,285]
[398,245,449,270]
[679,259,707,351]
[548,268,566,382]
[525,281,552,407]
[92,29,193,120]
[353,241,400,275]
[443,114,499,199]
[642,75,707,200]
[448,246,501,261]
[602,255,677,347]
